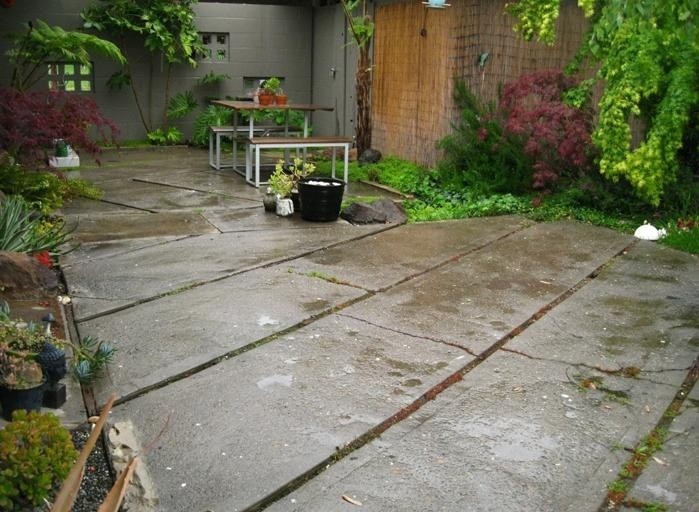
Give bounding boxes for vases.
[263,178,349,224]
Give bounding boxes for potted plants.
[0,297,71,424]
[266,156,317,217]
[253,75,288,108]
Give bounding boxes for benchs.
[231,135,353,192]
[205,122,306,172]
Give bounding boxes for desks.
[210,99,335,179]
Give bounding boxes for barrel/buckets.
[297,176,346,221]
